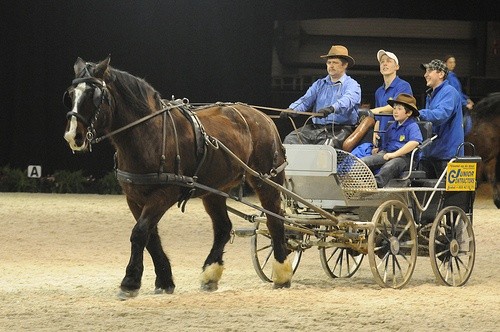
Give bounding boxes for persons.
[352,93,423,188]
[280,45,361,161]
[441,54,474,140]
[417,59,464,182]
[368,49,413,147]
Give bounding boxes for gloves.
[318,106,334,119]
[279,109,297,121]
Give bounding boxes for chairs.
[343,109,446,187]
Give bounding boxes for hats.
[421,59,449,75]
[387,93,420,118]
[376,49,399,66]
[319,45,355,65]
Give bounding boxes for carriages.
[63,53,482,302]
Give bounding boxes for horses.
[465,91,500,217]
[64,53,293,302]
